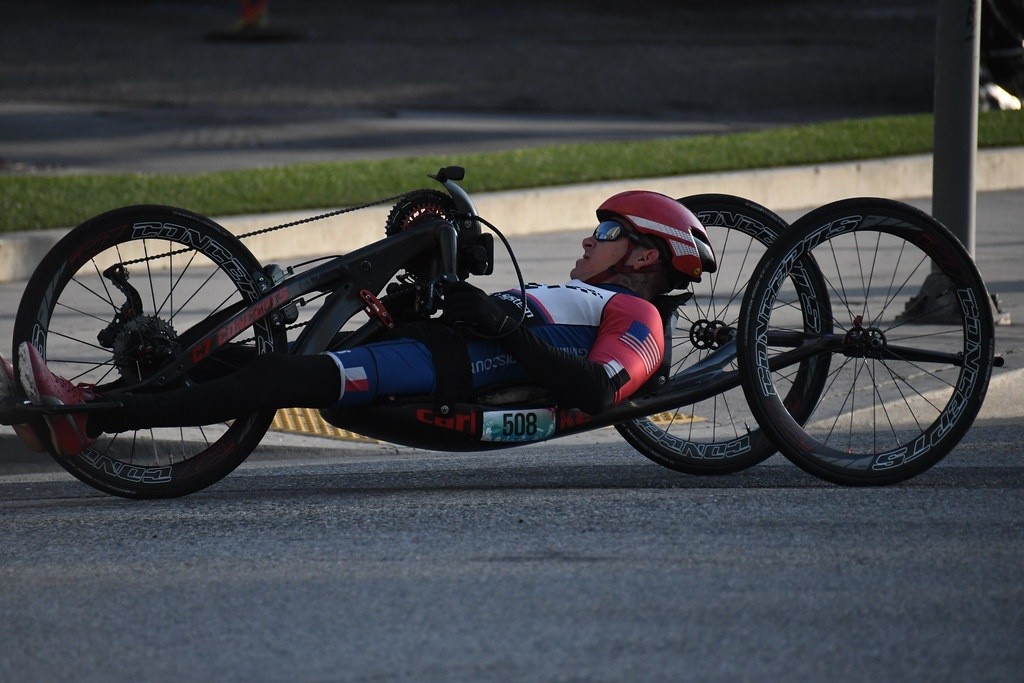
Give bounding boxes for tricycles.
[0,165,1006,501]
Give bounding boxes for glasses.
[594,219,660,256]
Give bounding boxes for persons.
[0,189,718,466]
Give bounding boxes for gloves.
[441,279,509,339]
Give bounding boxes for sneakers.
[19,341,99,456]
[0,352,49,454]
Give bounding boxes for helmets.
[596,190,718,290]
